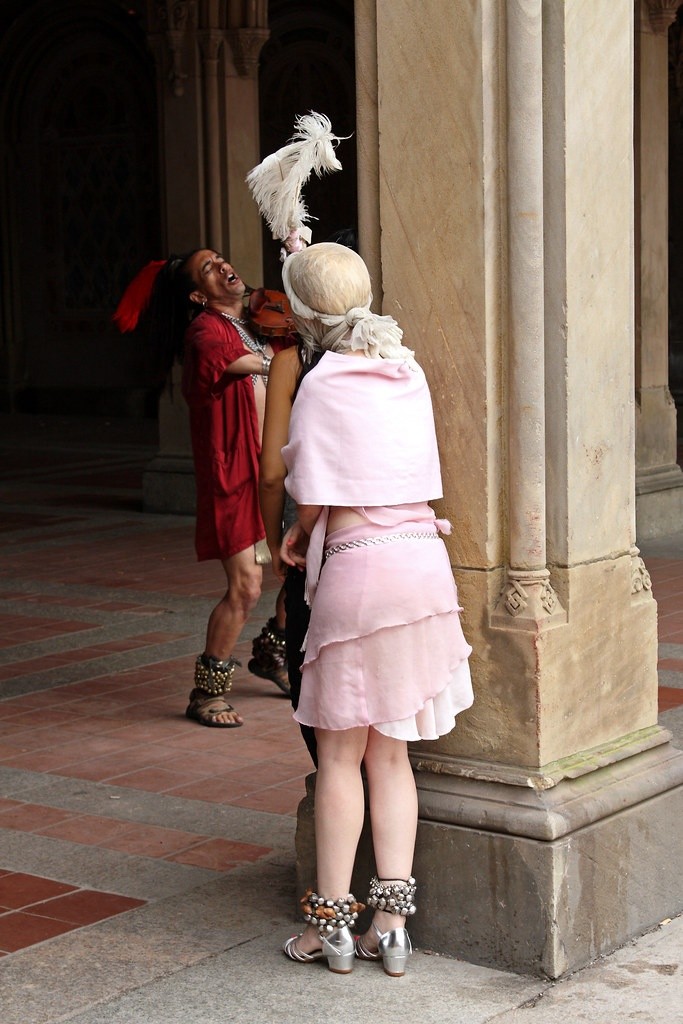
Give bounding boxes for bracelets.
[262,355,271,375]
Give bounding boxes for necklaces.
[221,312,268,355]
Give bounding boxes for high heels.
[282,925,355,973]
[356,921,413,977]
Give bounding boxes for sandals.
[186,687,243,727]
[247,646,291,695]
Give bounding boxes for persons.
[258,113,475,976]
[113,247,294,728]
